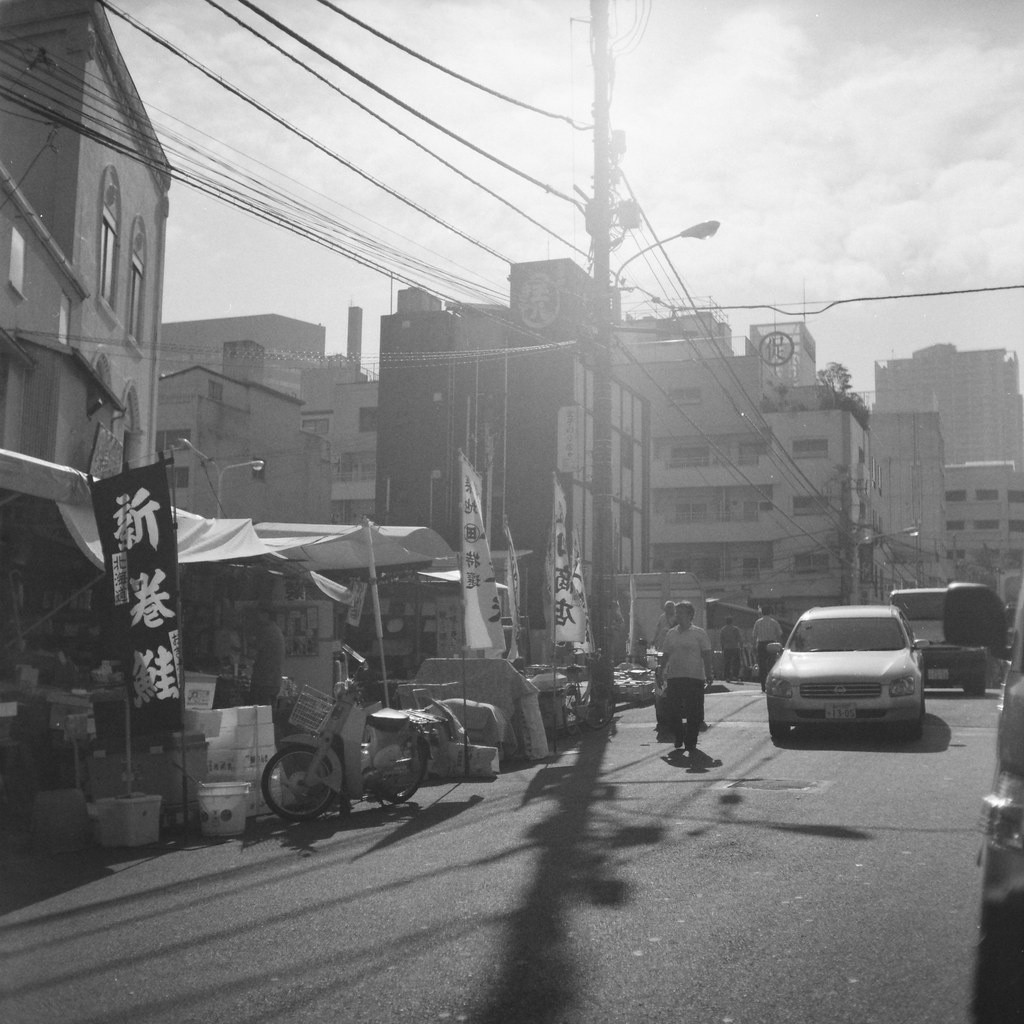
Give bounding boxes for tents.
[0,446,459,795]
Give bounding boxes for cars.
[943,581,1024,1024]
[763,604,926,745]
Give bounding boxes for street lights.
[852,527,919,604]
[177,437,264,520]
[590,219,720,729]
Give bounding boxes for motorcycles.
[260,646,439,825]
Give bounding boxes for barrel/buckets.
[197,781,248,837]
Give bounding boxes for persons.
[719,616,744,683]
[647,600,677,650]
[751,605,783,693]
[247,599,285,708]
[656,601,714,752]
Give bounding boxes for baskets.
[289,684,338,735]
[592,661,614,685]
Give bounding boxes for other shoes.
[684,744,696,758]
[674,734,684,748]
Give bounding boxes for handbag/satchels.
[650,680,670,725]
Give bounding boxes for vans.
[889,587,986,696]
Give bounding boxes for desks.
[1,678,138,799]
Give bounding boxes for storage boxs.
[33,669,287,848]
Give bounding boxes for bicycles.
[561,647,616,735]
